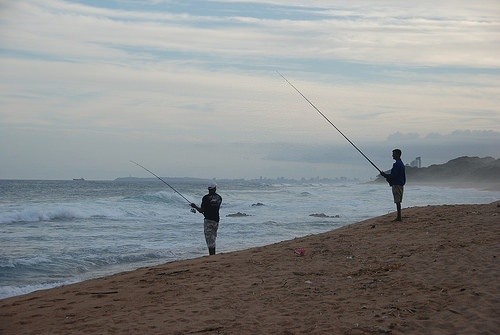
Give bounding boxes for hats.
[208,182,217,189]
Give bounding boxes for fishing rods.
[129,158,204,218]
[274,69,385,177]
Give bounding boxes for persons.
[381,148,406,221]
[191,184,222,255]
